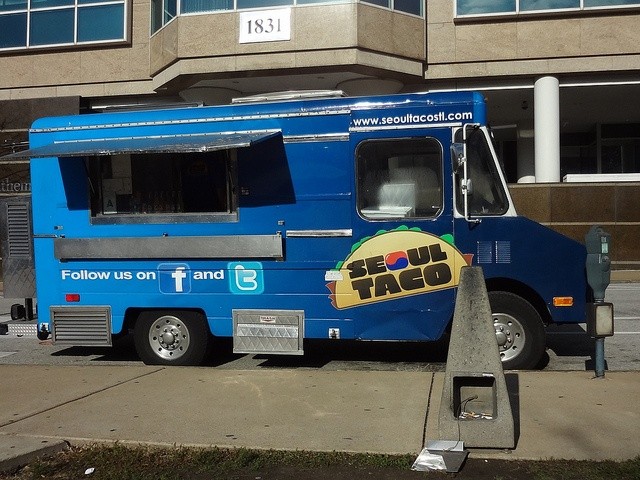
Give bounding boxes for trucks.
[29,89,595,369]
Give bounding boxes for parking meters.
[585,226,613,377]
